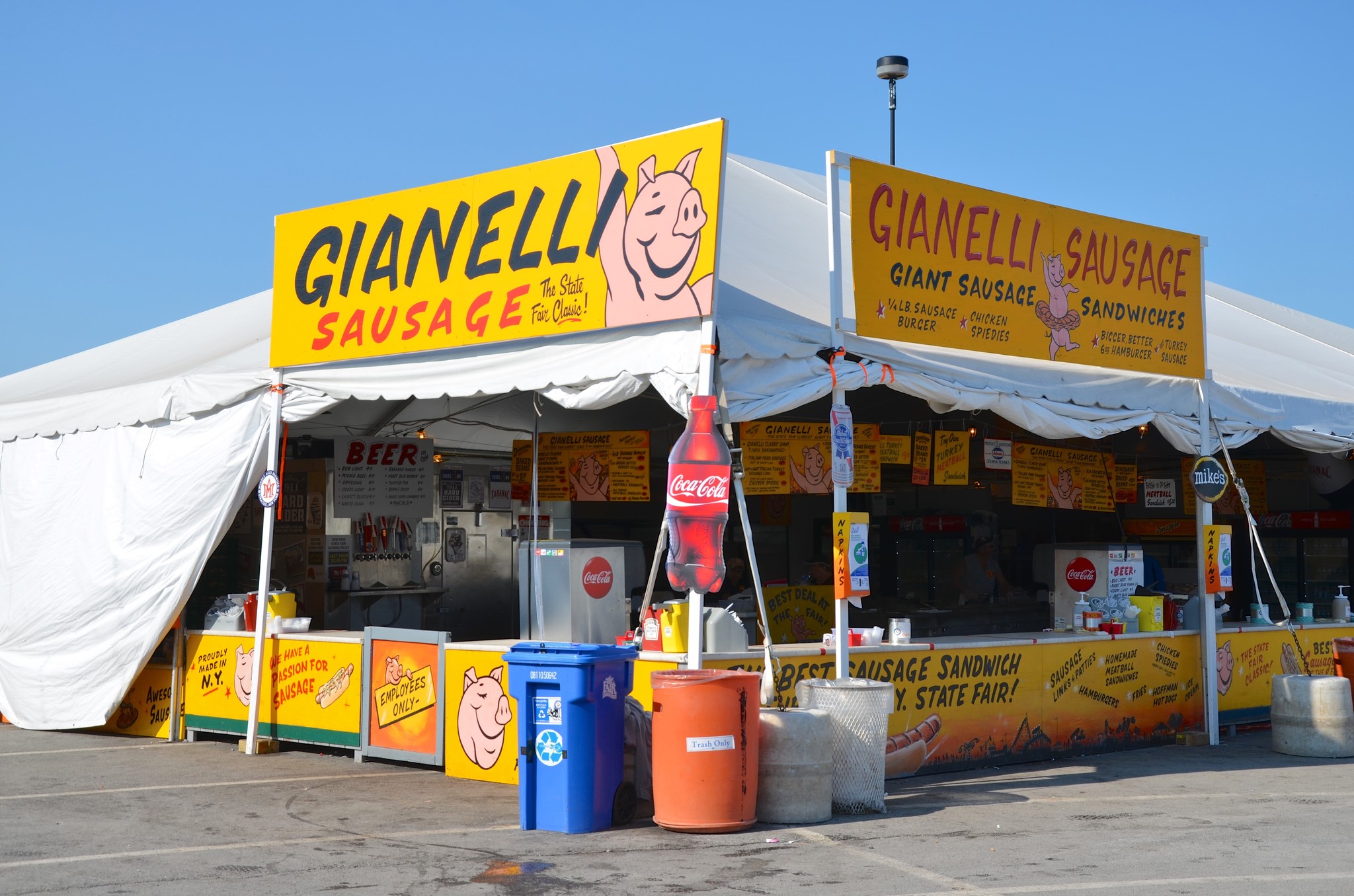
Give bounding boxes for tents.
[1,150,1354,754]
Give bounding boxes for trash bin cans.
[501,636,640,835]
[650,669,766,833]
[795,676,891,815]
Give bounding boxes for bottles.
[341,569,350,591]
[664,394,732,596]
[351,571,361,591]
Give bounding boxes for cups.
[1245,615,1251,623]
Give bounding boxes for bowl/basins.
[888,618,911,643]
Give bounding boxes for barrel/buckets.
[1082,610,1103,631]
[1250,603,1269,624]
[1295,601,1314,622]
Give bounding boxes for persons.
[1127,534,1167,593]
[953,536,1016,605]
[803,554,835,585]
[712,558,746,602]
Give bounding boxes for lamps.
[968,419,978,437]
[416,426,428,439]
[432,452,443,464]
[1138,422,1148,434]
